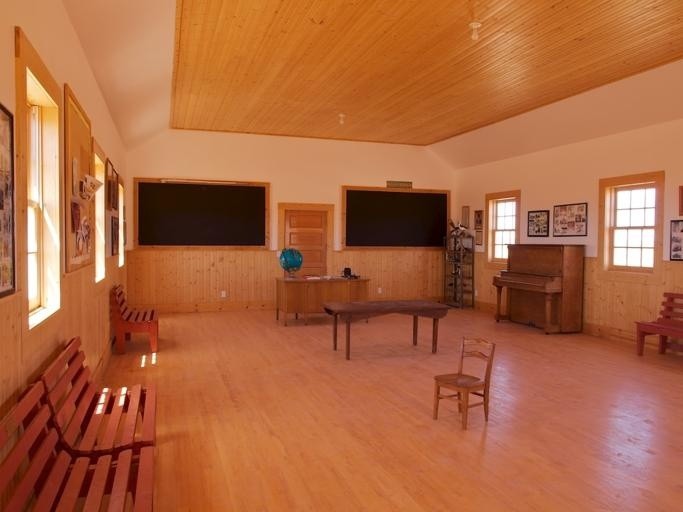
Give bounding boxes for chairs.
[431,335,497,431]
[110,288,160,353]
[634,292,682,357]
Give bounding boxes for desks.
[275,277,370,327]
[320,298,450,360]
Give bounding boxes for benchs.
[2,336,158,510]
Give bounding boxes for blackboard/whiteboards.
[342,186,451,250]
[133,178,271,251]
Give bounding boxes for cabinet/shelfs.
[441,236,476,310]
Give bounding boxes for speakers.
[344,268,351,277]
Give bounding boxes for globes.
[279,248,303,279]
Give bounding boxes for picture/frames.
[526,202,587,238]
[668,220,682,261]
[1,102,16,298]
[105,159,121,254]
